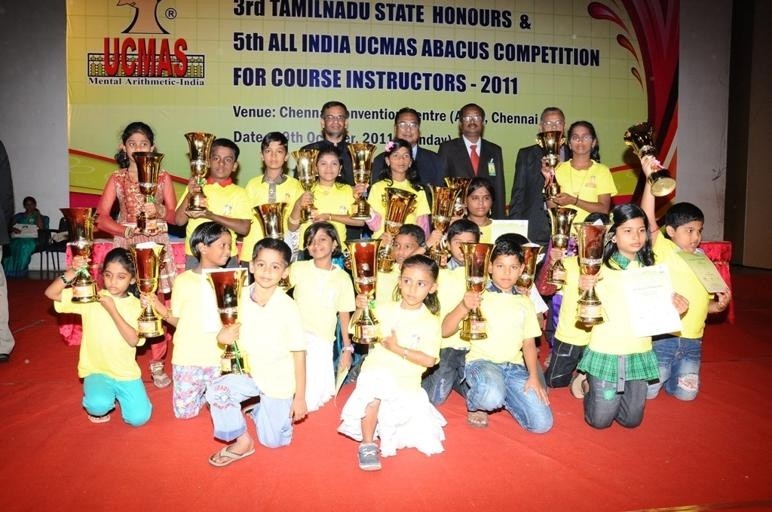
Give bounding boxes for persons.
[0,135,20,363]
[43,101,736,473]
[3,195,45,278]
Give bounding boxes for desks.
[696,240,734,323]
[56,232,246,350]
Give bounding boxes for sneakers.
[570,373,588,400]
[358,442,382,471]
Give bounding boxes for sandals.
[152,372,171,388]
[468,410,489,428]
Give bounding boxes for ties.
[469,145,481,176]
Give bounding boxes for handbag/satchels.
[11,223,39,238]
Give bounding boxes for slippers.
[208,443,255,466]
[88,414,111,423]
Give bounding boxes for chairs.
[46,217,70,274]
[4,216,51,272]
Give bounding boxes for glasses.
[460,115,482,123]
[541,120,564,129]
[396,122,419,128]
[325,115,347,122]
